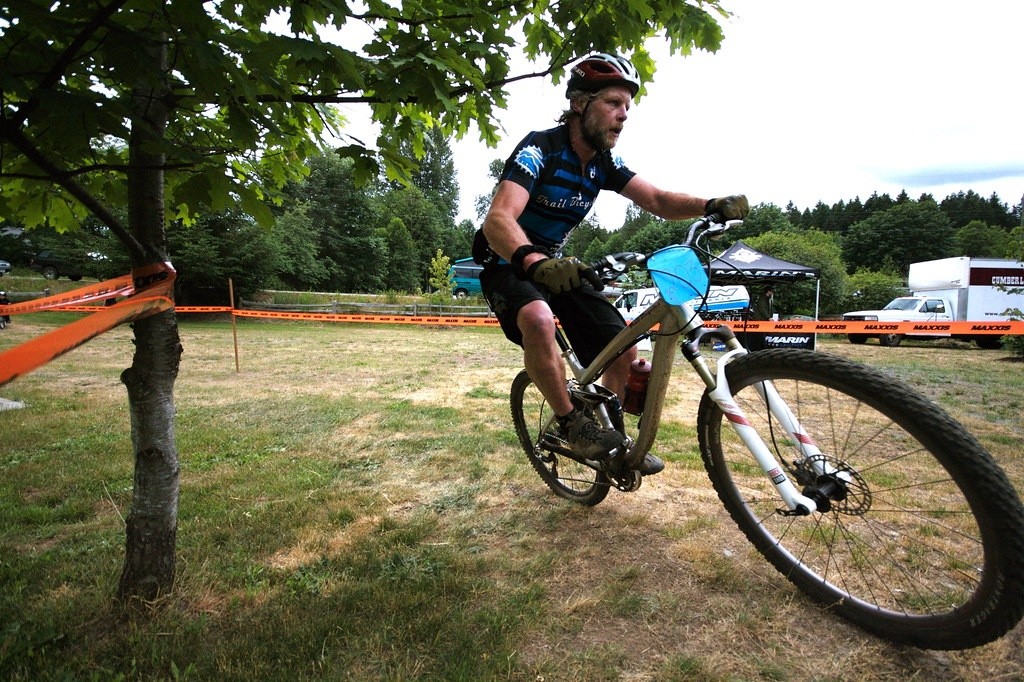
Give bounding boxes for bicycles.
[506,206,1023,652]
[0,291,11,330]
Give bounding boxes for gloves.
[527,258,604,294]
[704,195,749,219]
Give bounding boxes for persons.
[754,286,774,320]
[0,291,11,323]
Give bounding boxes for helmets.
[566,53,642,100]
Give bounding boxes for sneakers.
[630,443,665,475]
[565,415,623,460]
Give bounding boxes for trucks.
[610,284,750,341]
[842,256,1024,347]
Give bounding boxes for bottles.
[623,358,651,415]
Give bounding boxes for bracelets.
[511,245,552,282]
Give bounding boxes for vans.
[447,257,486,299]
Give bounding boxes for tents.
[702,241,822,350]
[479,52,749,477]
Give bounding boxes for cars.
[32,248,108,282]
[0,259,11,277]
[777,312,820,321]
